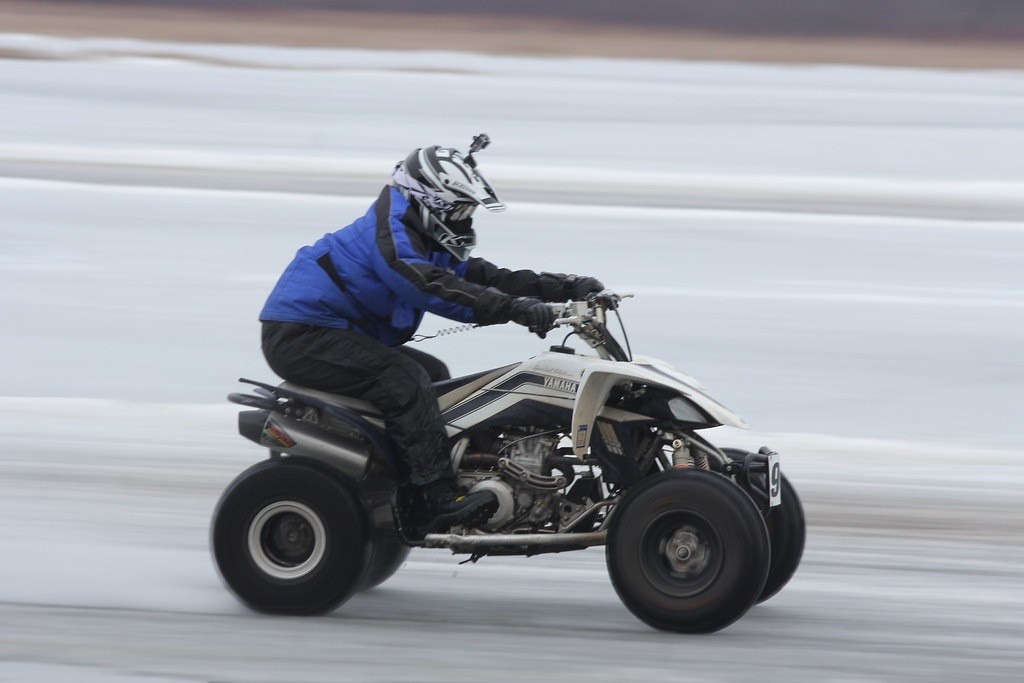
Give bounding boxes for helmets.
[392,145,506,262]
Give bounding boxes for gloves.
[565,274,605,308]
[507,296,553,339]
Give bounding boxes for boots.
[413,480,499,539]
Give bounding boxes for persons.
[258,146,604,530]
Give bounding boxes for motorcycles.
[207,292,808,636]
